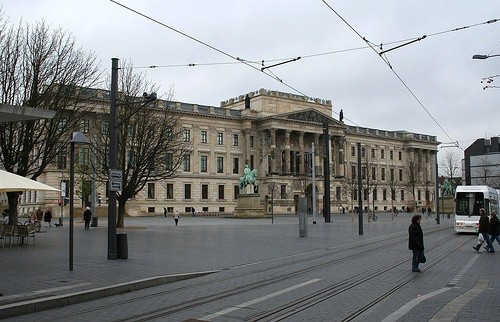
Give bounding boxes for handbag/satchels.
[477,233,485,244]
[419,255,427,263]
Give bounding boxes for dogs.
[54,223,60,228]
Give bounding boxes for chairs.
[0,220,36,248]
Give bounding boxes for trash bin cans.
[116,233,128,259]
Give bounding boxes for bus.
[454,186,500,234]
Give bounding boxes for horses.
[239,169,258,193]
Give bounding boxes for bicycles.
[1,212,41,233]
[368,213,377,222]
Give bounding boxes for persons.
[245,93,252,110]
[174,210,182,227]
[307,205,426,218]
[45,209,53,230]
[245,165,253,186]
[37,207,44,228]
[473,209,496,254]
[83,207,93,231]
[340,109,345,121]
[408,215,426,273]
[164,207,167,218]
[428,207,432,218]
[484,212,500,253]
[191,207,197,218]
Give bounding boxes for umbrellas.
[0,170,64,192]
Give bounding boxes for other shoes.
[473,246,480,251]
[484,247,489,251]
[488,249,496,253]
[413,269,420,272]
